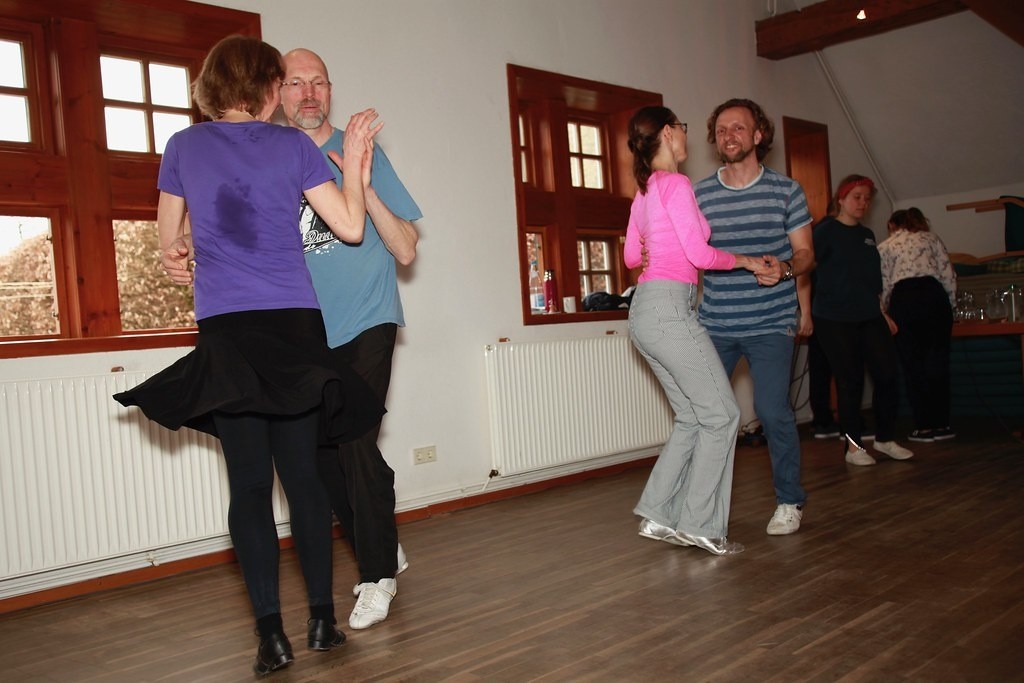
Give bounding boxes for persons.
[815,194,876,441]
[640,96,814,535]
[876,208,957,443]
[112,33,386,678]
[624,103,775,557]
[811,174,914,466]
[161,46,423,629]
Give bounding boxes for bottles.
[1008,283,1021,322]
[543,269,558,314]
[985,288,1008,319]
[529,260,546,314]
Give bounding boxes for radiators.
[485,335,675,477]
[0,374,293,581]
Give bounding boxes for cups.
[952,291,979,319]
[562,296,576,313]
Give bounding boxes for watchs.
[783,260,793,280]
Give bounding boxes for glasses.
[669,122,688,134]
[280,79,333,91]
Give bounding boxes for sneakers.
[348,578,398,629]
[353,542,408,596]
[767,503,803,535]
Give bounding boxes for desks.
[951,323,1024,445]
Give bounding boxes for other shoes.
[872,440,914,460]
[813,423,840,438]
[906,429,935,442]
[675,527,746,556]
[845,449,877,466]
[935,428,957,440]
[840,428,876,441]
[637,517,689,547]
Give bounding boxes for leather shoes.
[307,616,346,650]
[252,625,295,677]
